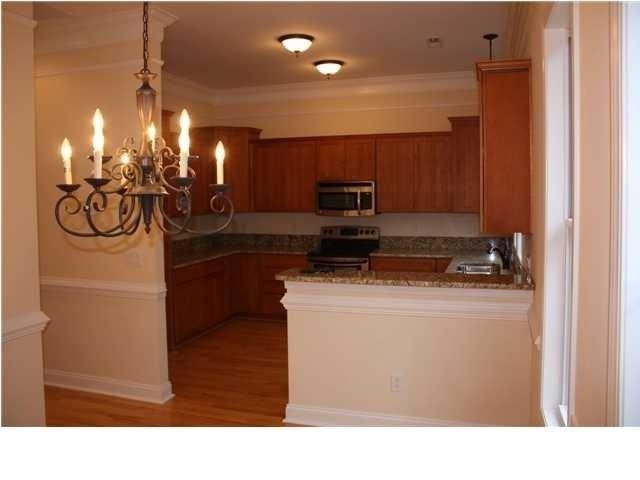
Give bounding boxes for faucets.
[487,247,506,269]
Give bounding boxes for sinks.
[455,263,494,274]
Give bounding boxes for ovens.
[306,258,371,272]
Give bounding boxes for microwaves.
[314,180,376,218]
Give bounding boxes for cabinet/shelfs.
[371,258,452,271]
[166,254,237,350]
[448,116,480,214]
[238,254,309,320]
[164,124,451,212]
[474,60,532,234]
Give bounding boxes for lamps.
[54,2,235,239]
[277,33,345,80]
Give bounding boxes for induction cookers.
[306,225,380,258]
